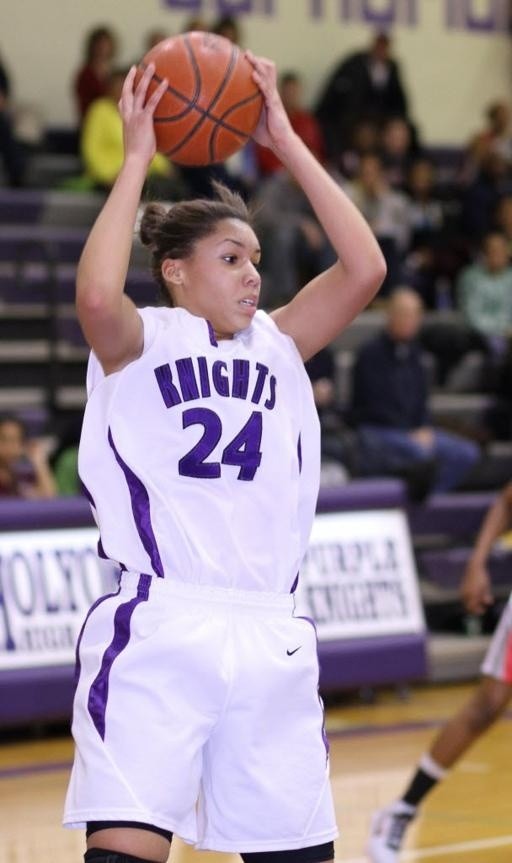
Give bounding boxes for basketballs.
[133,33,266,170]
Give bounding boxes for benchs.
[0,126,512,465]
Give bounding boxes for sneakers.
[367,799,417,862]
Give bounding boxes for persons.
[364,470,512,860]
[0,9,512,501]
[62,46,390,863]
